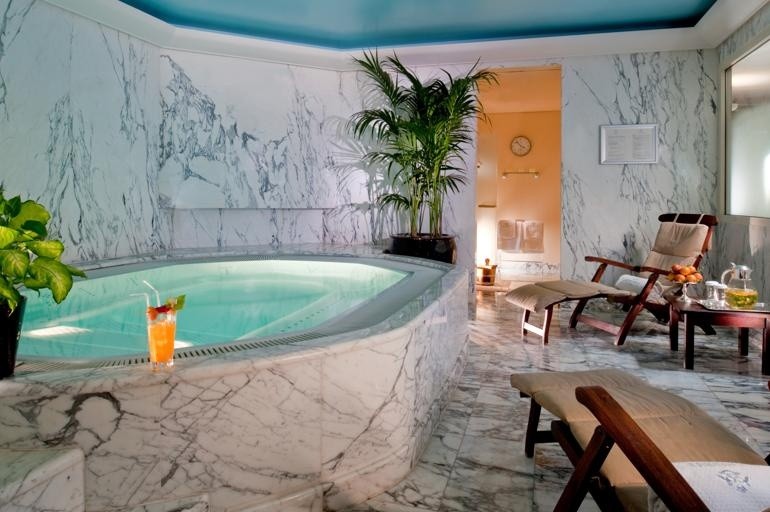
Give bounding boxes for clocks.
[510,136,532,156]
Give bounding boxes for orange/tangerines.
[665,263,703,283]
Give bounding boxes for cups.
[146,310,176,371]
[706,280,726,307]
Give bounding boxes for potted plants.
[0,186,86,382]
[345,46,499,264]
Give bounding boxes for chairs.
[510,367,770,512]
[504,212,719,348]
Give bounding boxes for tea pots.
[721,261,758,308]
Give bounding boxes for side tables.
[654,283,770,376]
